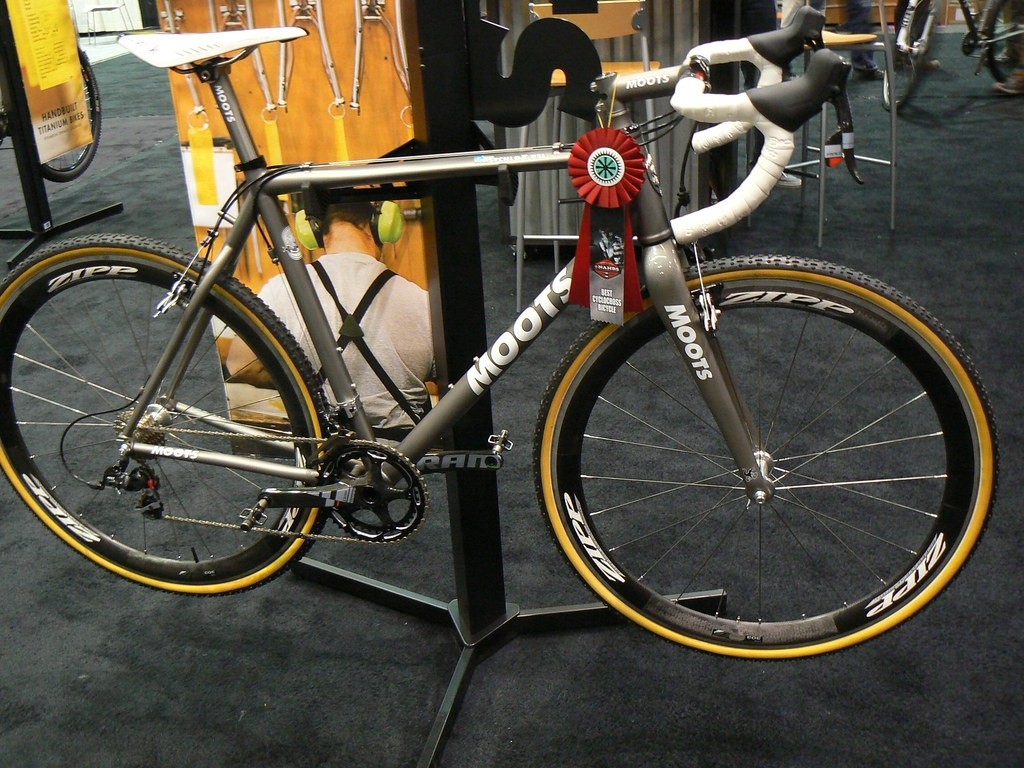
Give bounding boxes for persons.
[892,0,940,69]
[992,0,1024,96]
[226,198,438,431]
[808,0,885,81]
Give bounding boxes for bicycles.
[0,0,1001,673]
[880,0,1024,110]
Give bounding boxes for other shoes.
[898,59,938,72]
[853,66,894,79]
[993,73,1024,95]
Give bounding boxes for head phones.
[295,202,405,250]
